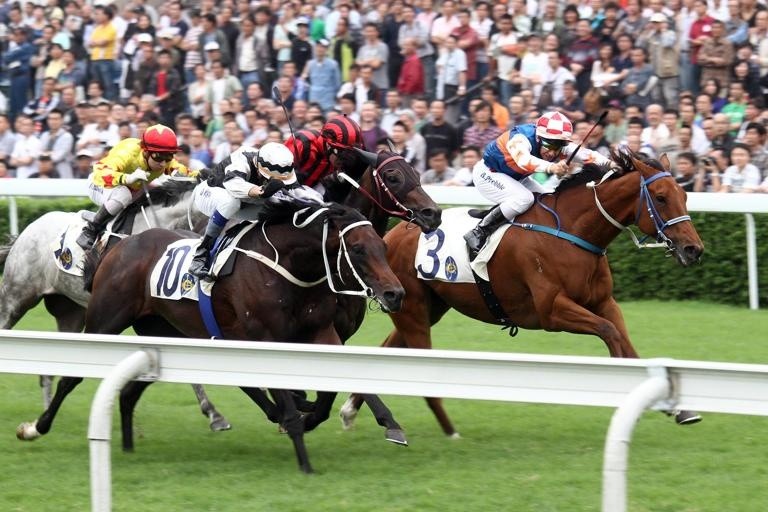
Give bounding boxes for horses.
[339,145,703,439]
[239,139,443,432]
[0,168,231,432]
[16,203,406,473]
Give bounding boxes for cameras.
[701,157,716,169]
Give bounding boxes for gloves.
[258,179,288,199]
[124,168,151,184]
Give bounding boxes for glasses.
[539,139,565,150]
[328,148,345,157]
[147,151,174,162]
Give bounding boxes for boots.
[76,203,117,251]
[463,205,510,253]
[188,234,220,278]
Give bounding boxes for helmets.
[535,111,574,147]
[138,123,183,154]
[320,113,366,152]
[252,141,298,186]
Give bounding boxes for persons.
[187,142,324,278]
[0,1,768,194]
[283,113,367,189]
[463,109,624,259]
[75,123,200,251]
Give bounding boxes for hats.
[315,38,330,47]
[649,12,669,24]
[136,32,154,45]
[295,16,310,27]
[156,30,175,40]
[203,40,220,51]
